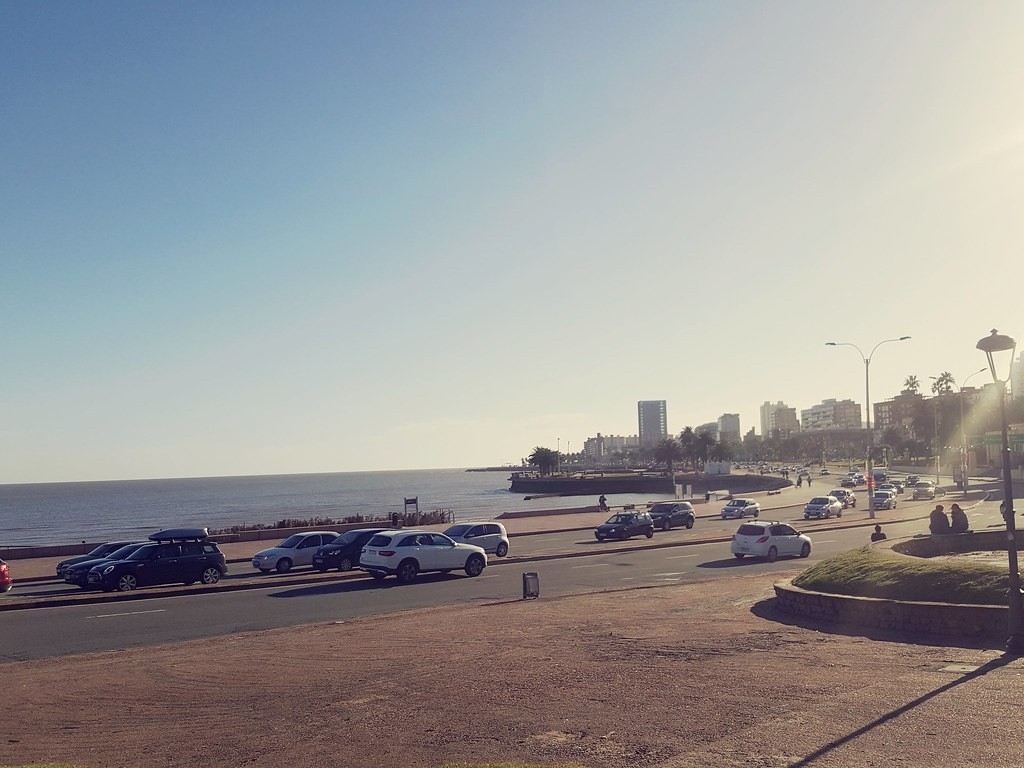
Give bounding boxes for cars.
[874,491,897,510]
[803,495,843,519]
[359,530,488,585]
[828,489,857,510]
[720,498,760,520]
[56,528,229,592]
[442,522,509,558]
[252,531,340,573]
[730,520,812,561]
[312,527,398,572]
[647,501,696,530]
[737,459,920,497]
[594,510,655,541]
[913,481,936,500]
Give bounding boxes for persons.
[871,525,886,542]
[807,476,812,487]
[798,475,802,487]
[928,505,949,535]
[599,494,607,510]
[951,504,968,532]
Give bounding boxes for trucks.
[873,467,889,490]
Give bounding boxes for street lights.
[927,367,987,498]
[825,336,912,520]
[976,329,1024,655]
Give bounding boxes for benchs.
[205,534,241,542]
